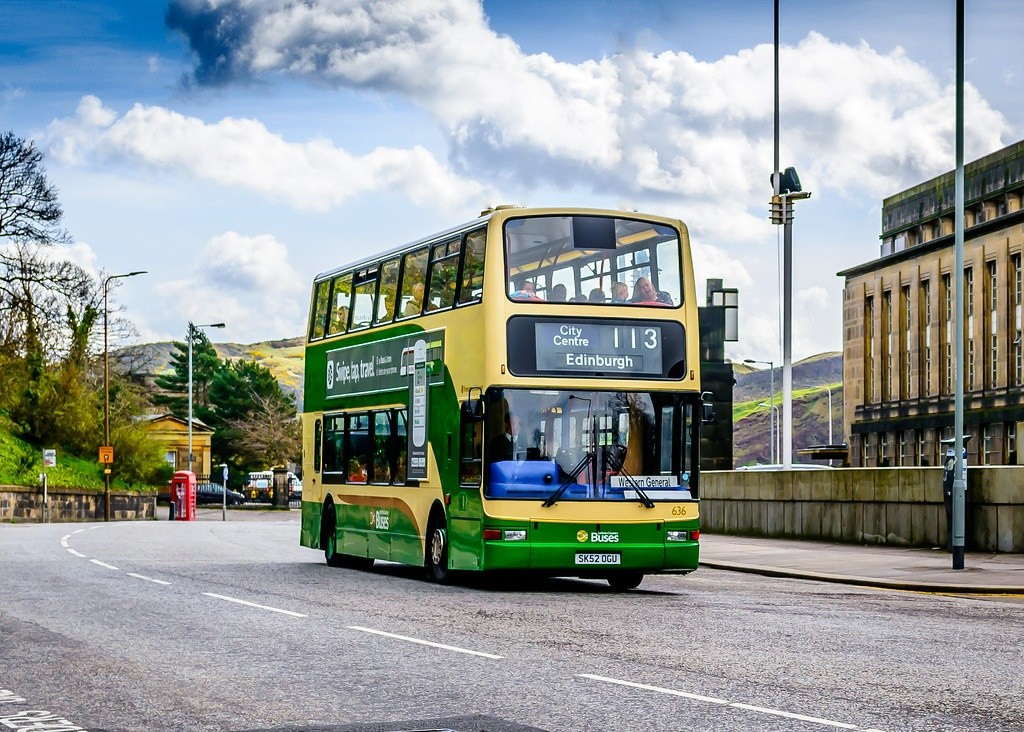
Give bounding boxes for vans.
[249,471,302,502]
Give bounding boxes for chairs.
[331,436,627,482]
[354,297,632,329]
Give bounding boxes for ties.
[510,436,515,443]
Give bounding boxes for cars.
[234,479,274,502]
[195,483,245,506]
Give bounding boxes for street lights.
[758,402,780,465]
[802,384,832,466]
[219,464,228,521]
[744,360,774,467]
[771,167,810,469]
[187,323,226,471]
[104,271,148,521]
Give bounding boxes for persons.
[516,281,605,303]
[380,295,404,322]
[349,450,407,483]
[632,277,674,306]
[330,305,356,333]
[503,411,530,461]
[404,283,437,315]
[611,282,630,303]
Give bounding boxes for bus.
[300,204,716,591]
